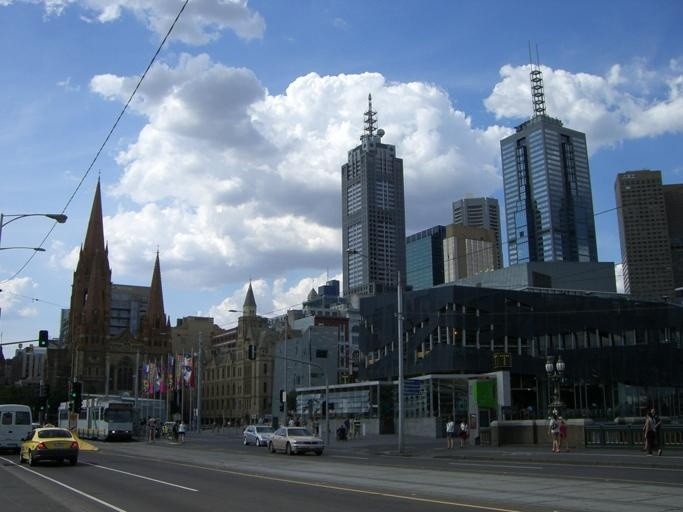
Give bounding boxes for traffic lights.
[39,331,48,347]
[40,404,50,411]
[69,383,82,413]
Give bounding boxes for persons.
[547,414,560,453]
[558,419,568,451]
[458,418,468,449]
[643,411,661,456]
[642,408,661,451]
[146,417,186,443]
[446,415,455,450]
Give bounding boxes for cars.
[19,428,79,466]
[243,425,324,456]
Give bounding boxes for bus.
[58,398,132,442]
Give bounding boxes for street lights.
[344,249,404,452]
[229,310,288,426]
[0,212,67,251]
[545,355,567,407]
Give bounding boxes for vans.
[0,404,32,454]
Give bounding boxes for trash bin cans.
[491,421,507,446]
